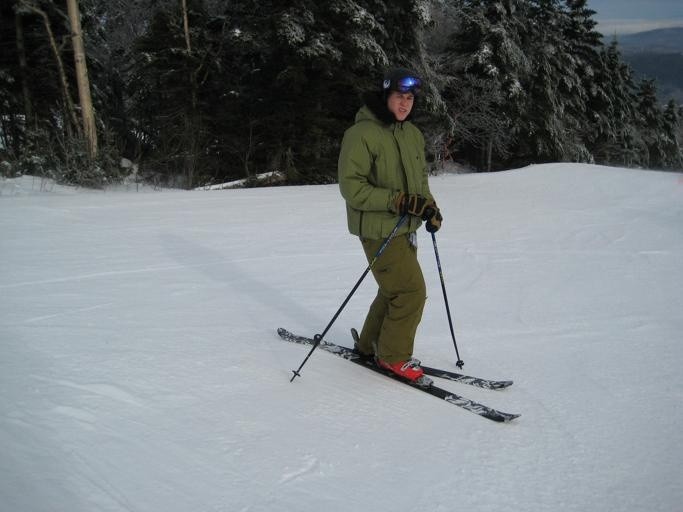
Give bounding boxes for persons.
[334,68,444,383]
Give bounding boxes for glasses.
[396,75,422,93]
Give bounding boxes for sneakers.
[377,356,426,383]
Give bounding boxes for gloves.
[424,204,443,233]
[398,193,436,220]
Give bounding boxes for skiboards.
[277,327,521,422]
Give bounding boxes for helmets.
[380,67,423,99]
